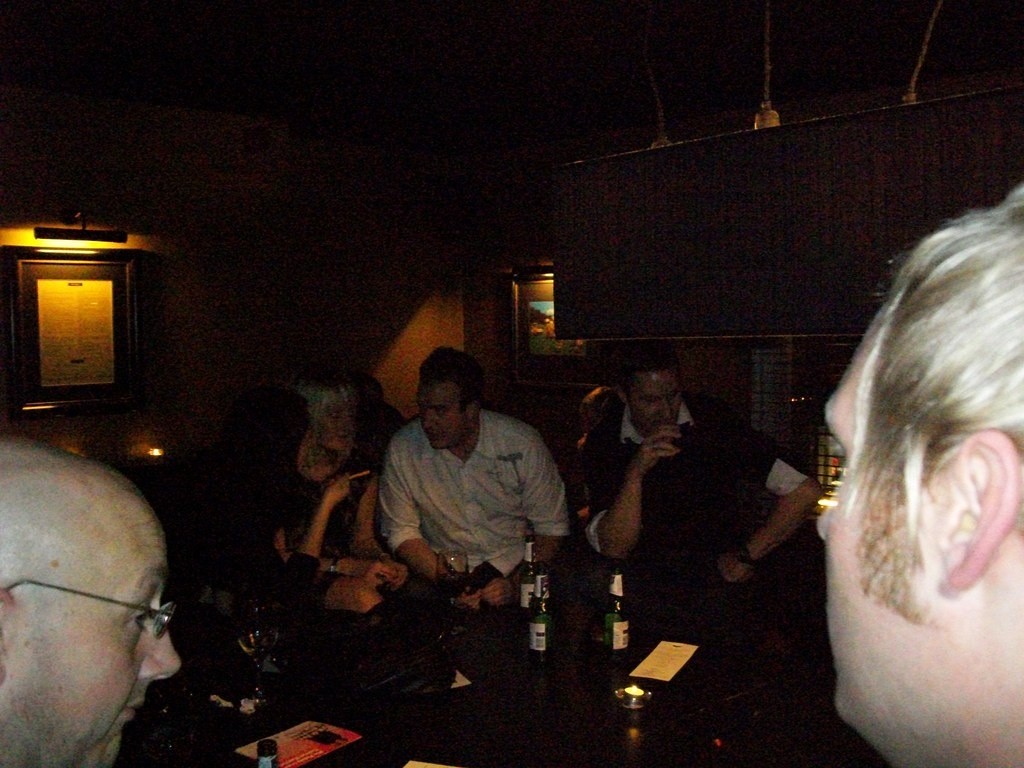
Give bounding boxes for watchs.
[735,545,754,566]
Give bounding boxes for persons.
[0,170,1024,768]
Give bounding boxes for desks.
[111,550,888,768]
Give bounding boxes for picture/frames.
[508,266,607,390]
[0,246,143,422]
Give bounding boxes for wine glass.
[436,548,473,624]
[234,601,280,707]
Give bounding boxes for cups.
[136,686,203,763]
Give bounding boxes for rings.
[653,441,659,449]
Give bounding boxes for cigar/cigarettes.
[349,469,371,480]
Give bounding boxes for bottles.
[519,532,541,616]
[529,574,553,664]
[604,566,630,657]
[253,739,279,768]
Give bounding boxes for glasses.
[4,578,177,637]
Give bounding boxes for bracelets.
[328,554,341,578]
